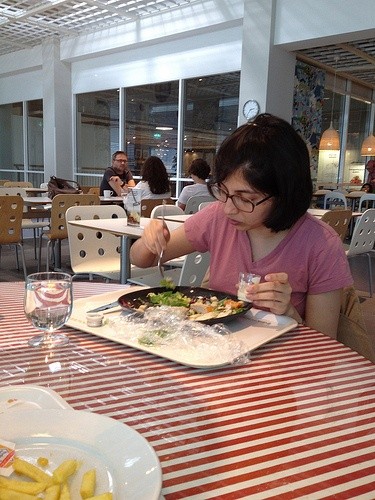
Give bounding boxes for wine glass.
[24,272,73,347]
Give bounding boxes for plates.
[116,284,253,326]
[1,410,163,499]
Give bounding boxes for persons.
[125,156,173,214]
[177,160,218,210]
[100,151,136,198]
[132,113,353,339]
[362,160,375,194]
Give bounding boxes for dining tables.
[0,279,375,500]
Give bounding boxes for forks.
[158,200,167,279]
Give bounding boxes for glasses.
[207,177,273,213]
[115,159,127,162]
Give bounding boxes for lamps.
[318,69,340,150]
[360,87,375,157]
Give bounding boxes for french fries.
[0,456,115,500]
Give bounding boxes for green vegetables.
[148,276,191,309]
[214,298,249,314]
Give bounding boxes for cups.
[236,271,261,303]
[86,314,104,326]
[104,190,114,198]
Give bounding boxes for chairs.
[0,180,375,297]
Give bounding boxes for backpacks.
[47,175,82,200]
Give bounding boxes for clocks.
[243,99,259,120]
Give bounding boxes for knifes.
[86,300,119,315]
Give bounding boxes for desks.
[21,187,47,196]
[67,216,185,284]
[24,196,124,270]
[307,208,363,220]
[158,214,194,223]
